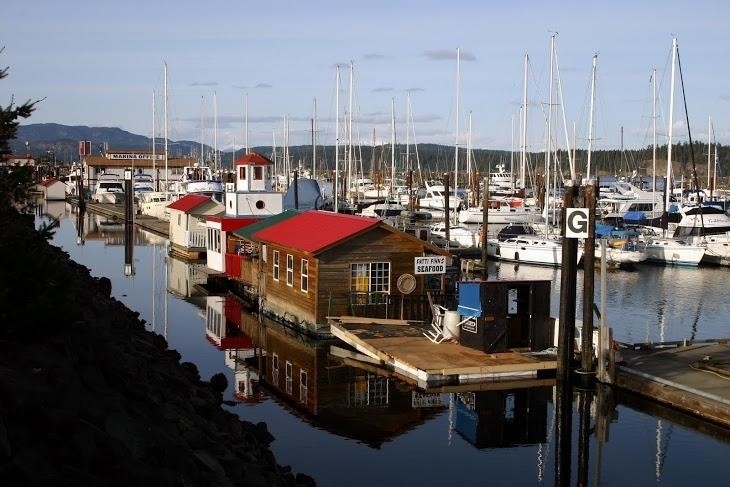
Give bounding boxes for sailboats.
[64,57,227,206]
[268,31,730,270]
